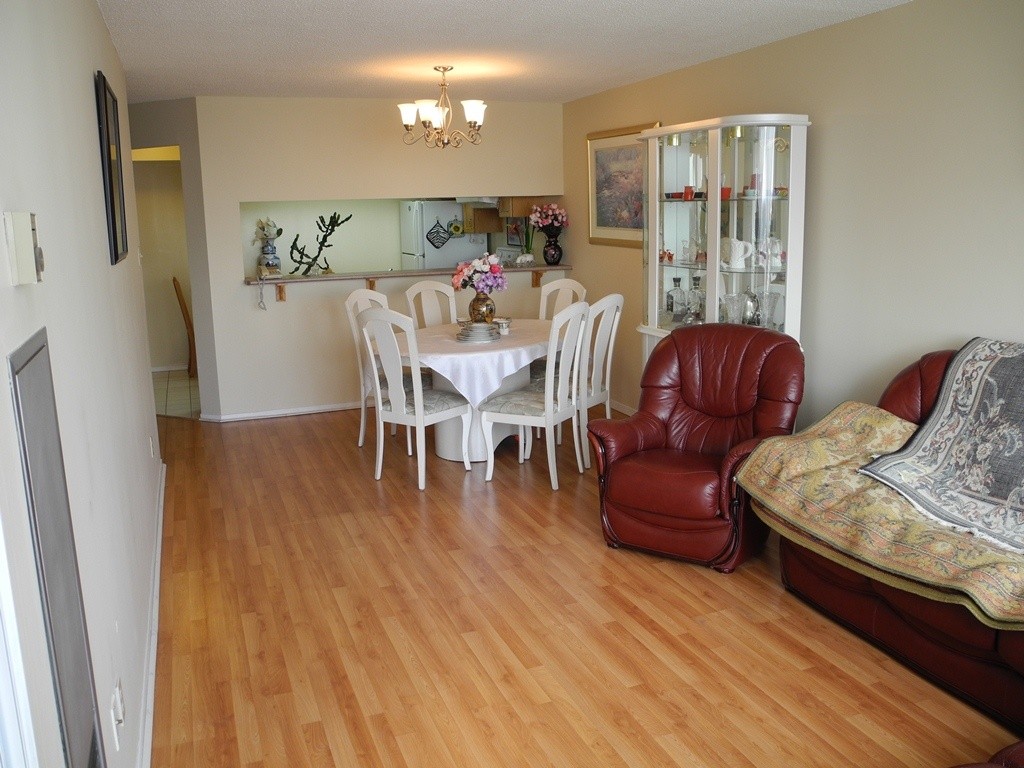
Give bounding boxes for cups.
[684,186,694,200]
[757,293,779,330]
[497,320,510,336]
[721,187,731,199]
[724,293,745,325]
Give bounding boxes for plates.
[456,322,501,342]
[494,316,511,322]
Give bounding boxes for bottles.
[687,276,706,322]
[665,278,687,323]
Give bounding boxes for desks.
[370,317,567,466]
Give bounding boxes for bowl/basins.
[665,193,683,198]
[457,318,472,327]
[694,192,704,197]
[745,189,755,196]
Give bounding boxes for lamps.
[729,125,745,138]
[397,65,488,150]
[666,133,681,146]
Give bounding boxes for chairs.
[172,277,197,377]
[346,280,625,492]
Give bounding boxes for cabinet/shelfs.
[497,197,543,218]
[463,203,502,233]
[637,113,812,436]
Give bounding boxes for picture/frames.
[95,69,129,267]
[507,219,527,246]
[584,120,662,248]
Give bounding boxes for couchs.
[586,322,805,575]
[778,350,1023,768]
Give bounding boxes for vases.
[469,293,496,323]
[543,240,564,265]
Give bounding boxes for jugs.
[728,239,752,269]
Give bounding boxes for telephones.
[256,265,283,279]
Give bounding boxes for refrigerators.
[400,200,488,270]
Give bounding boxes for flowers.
[505,219,534,252]
[529,203,569,242]
[452,251,509,294]
